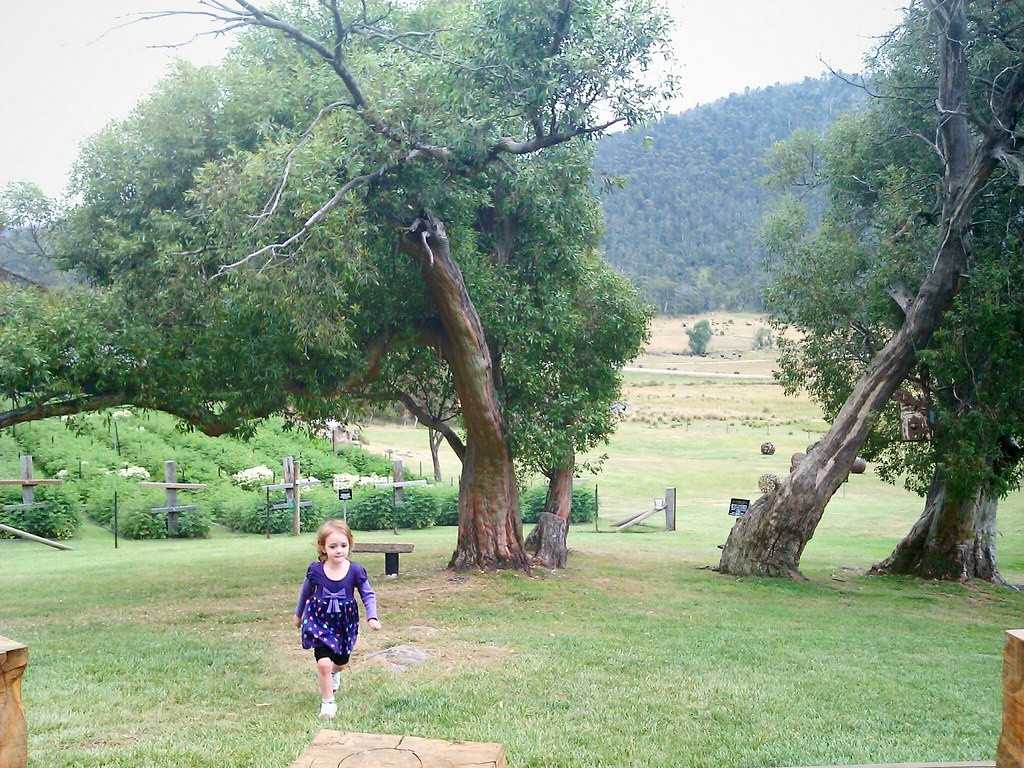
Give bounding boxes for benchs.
[352,543,414,575]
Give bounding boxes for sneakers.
[331,669,340,691]
[318,702,337,718]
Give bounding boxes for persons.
[293,519,382,720]
